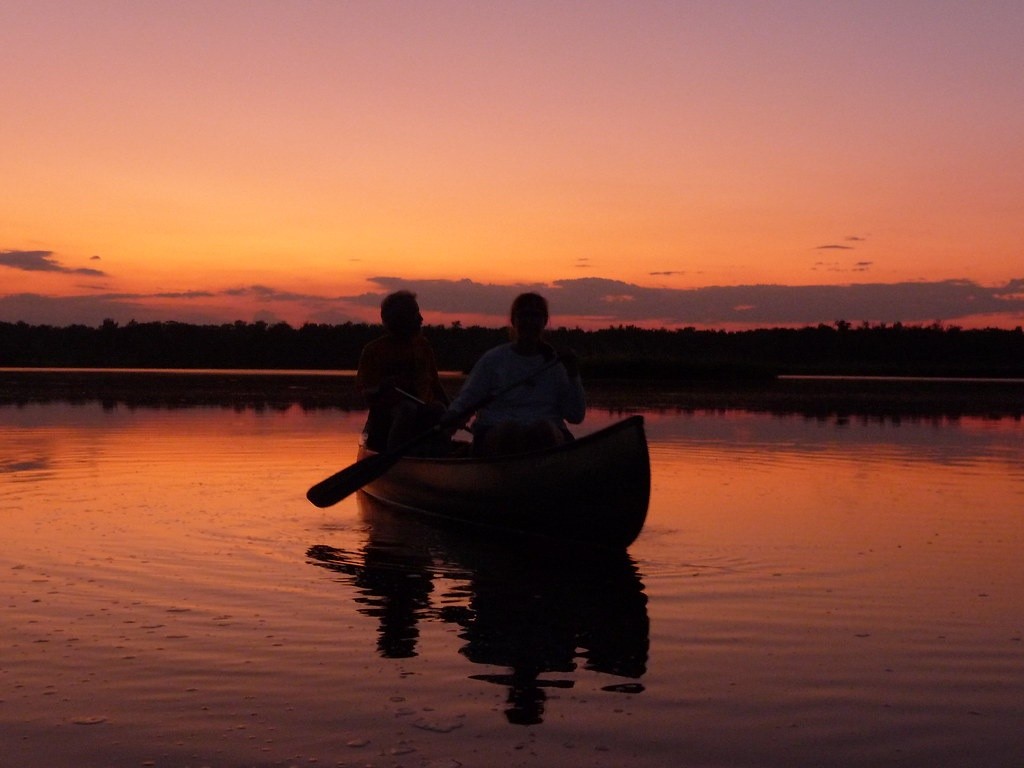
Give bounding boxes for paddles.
[392,386,473,434]
[307,359,560,508]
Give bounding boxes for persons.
[354,289,451,456]
[440,291,586,457]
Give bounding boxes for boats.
[357,413,651,554]
